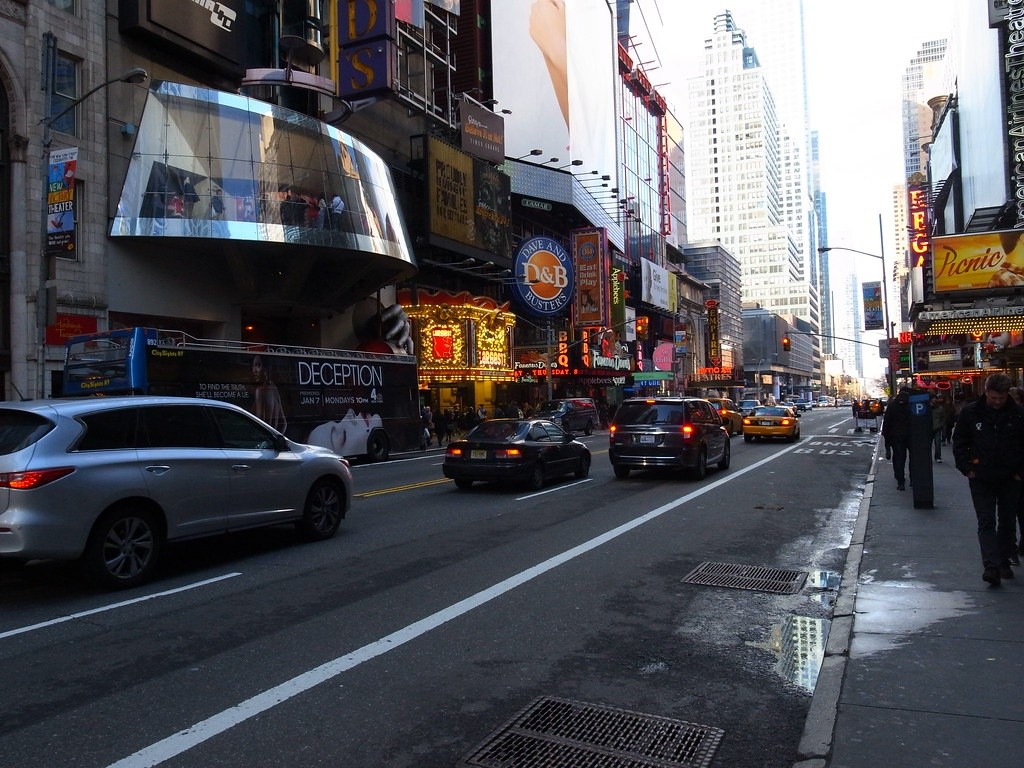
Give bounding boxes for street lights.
[817,245,897,402]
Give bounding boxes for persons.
[280,195,293,225]
[294,194,308,226]
[952,374,1024,585]
[930,393,942,463]
[307,195,320,228]
[529,1,568,132]
[210,189,226,220]
[881,387,913,490]
[182,177,200,219]
[941,396,954,446]
[317,194,328,229]
[251,355,287,440]
[307,408,383,457]
[1008,389,1024,565]
[420,400,524,446]
[330,193,345,231]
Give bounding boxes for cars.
[859,399,881,417]
[0,397,352,585]
[775,396,852,411]
[742,405,801,444]
[704,397,744,438]
[442,417,592,489]
[735,400,761,418]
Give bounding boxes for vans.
[608,396,730,480]
[538,398,601,436]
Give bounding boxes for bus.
[57,324,421,465]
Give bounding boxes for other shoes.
[984,562,1015,587]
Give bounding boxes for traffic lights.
[782,337,791,354]
